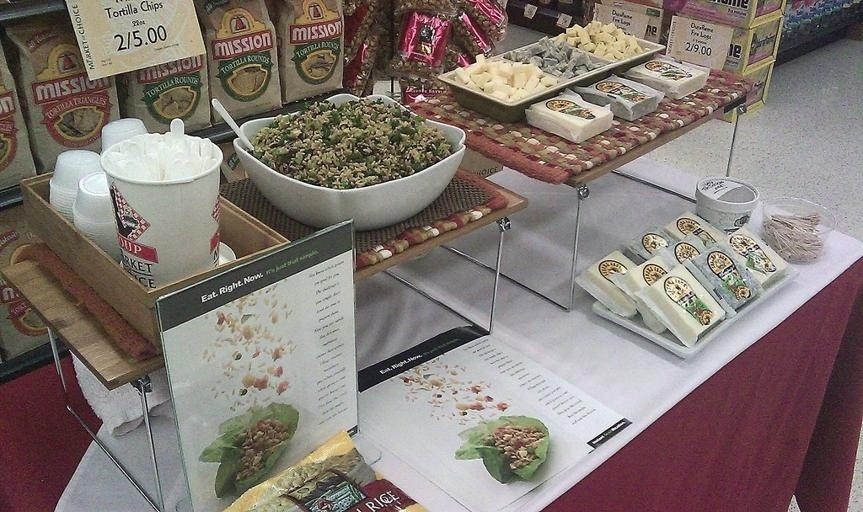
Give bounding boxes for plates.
[439,34,666,121]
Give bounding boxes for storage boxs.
[666,0,787,123]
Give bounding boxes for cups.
[696,175,758,235]
[761,194,837,263]
[48,118,149,263]
[100,133,225,290]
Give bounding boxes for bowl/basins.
[234,91,465,230]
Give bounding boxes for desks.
[0,169,527,512]
[0,160,863,512]
[406,56,763,312]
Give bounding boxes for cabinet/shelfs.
[0,0,351,386]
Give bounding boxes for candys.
[455,19,649,103]
